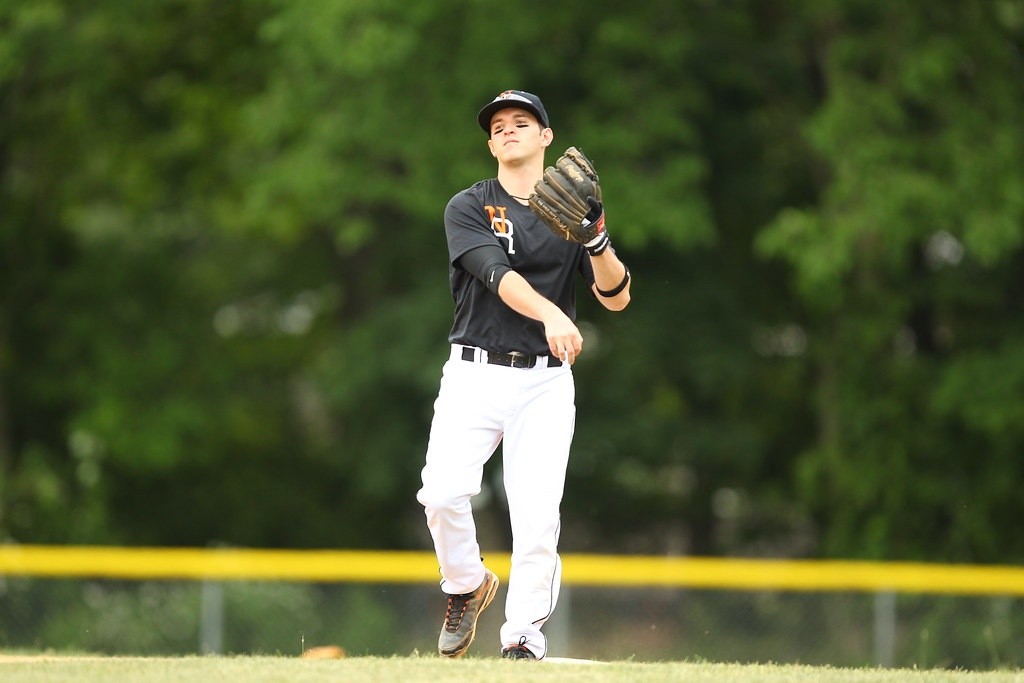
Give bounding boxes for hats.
[476,89,550,134]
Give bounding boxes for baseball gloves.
[527,145,605,242]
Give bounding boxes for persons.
[416,90,632,661]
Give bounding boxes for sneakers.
[501,643,536,661]
[437,568,500,660]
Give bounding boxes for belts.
[463,345,566,370]
